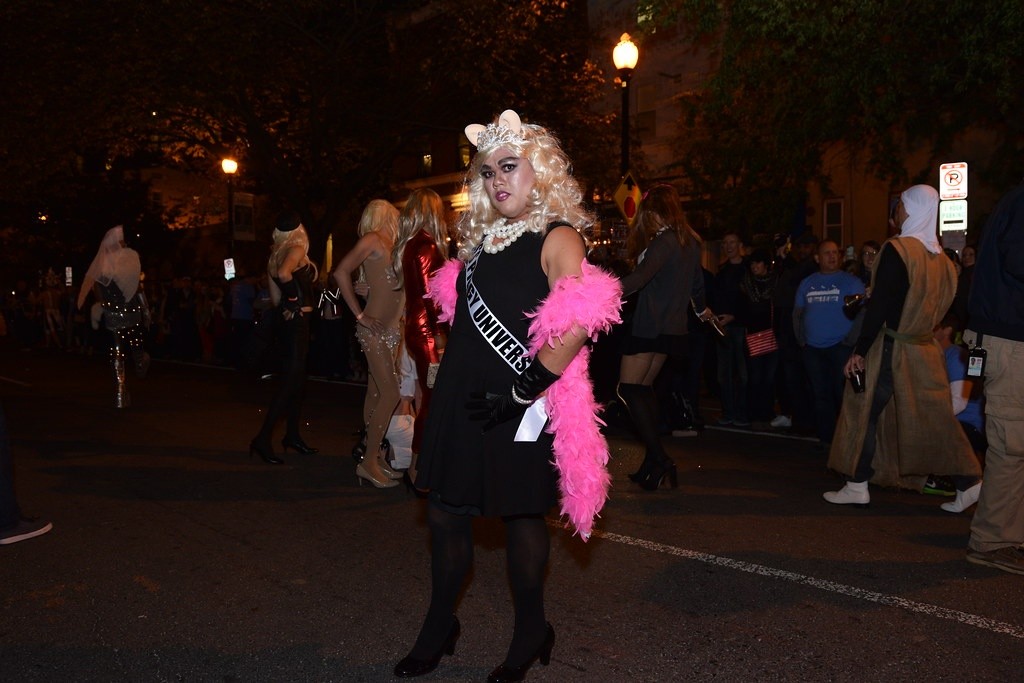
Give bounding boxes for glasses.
[862,251,878,256]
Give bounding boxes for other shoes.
[718,413,736,424]
[733,414,753,425]
[117,393,131,408]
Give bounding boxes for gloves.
[463,355,561,433]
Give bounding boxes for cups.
[847,368,866,393]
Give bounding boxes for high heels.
[403,469,430,499]
[355,457,403,489]
[249,438,284,464]
[394,615,459,676]
[487,622,556,683]
[282,436,319,454]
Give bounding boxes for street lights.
[220,157,237,327]
[612,31,638,185]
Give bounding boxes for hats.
[794,233,819,244]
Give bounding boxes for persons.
[383,186,450,497]
[710,233,980,439]
[966,168,1024,577]
[821,183,985,514]
[0,399,54,545]
[248,208,321,465]
[395,108,628,683]
[620,184,713,490]
[0,276,231,368]
[331,198,405,489]
[91,226,150,408]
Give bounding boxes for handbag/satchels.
[745,299,779,357]
[426,362,441,389]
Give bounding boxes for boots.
[608,383,677,490]
[672,401,698,436]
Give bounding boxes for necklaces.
[481,217,531,255]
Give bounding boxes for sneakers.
[965,545,1024,575]
[823,481,870,504]
[770,415,793,427]
[0,517,53,544]
[923,474,957,496]
[941,479,981,512]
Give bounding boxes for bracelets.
[512,386,532,404]
[356,311,364,320]
